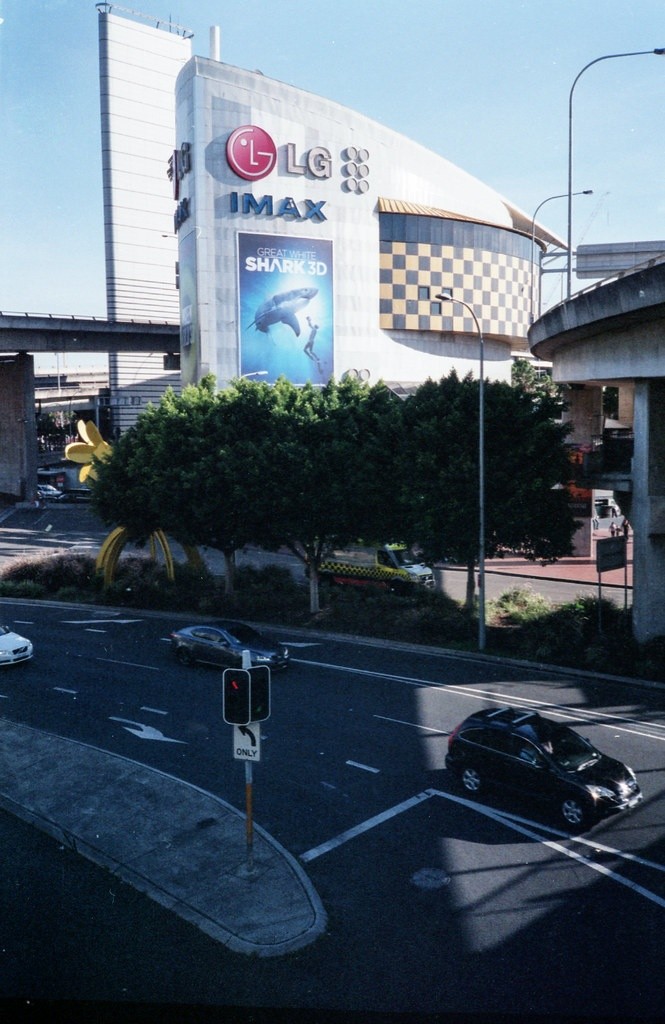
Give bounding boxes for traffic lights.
[245,665,272,723]
[221,667,251,727]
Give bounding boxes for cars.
[0,624,35,666]
[168,620,291,674]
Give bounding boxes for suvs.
[443,705,644,827]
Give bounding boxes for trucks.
[303,543,435,597]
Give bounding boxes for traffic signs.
[232,723,262,763]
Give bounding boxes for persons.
[302,316,321,361]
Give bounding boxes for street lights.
[68,388,82,444]
[526,189,595,322]
[564,46,665,301]
[435,293,489,651]
